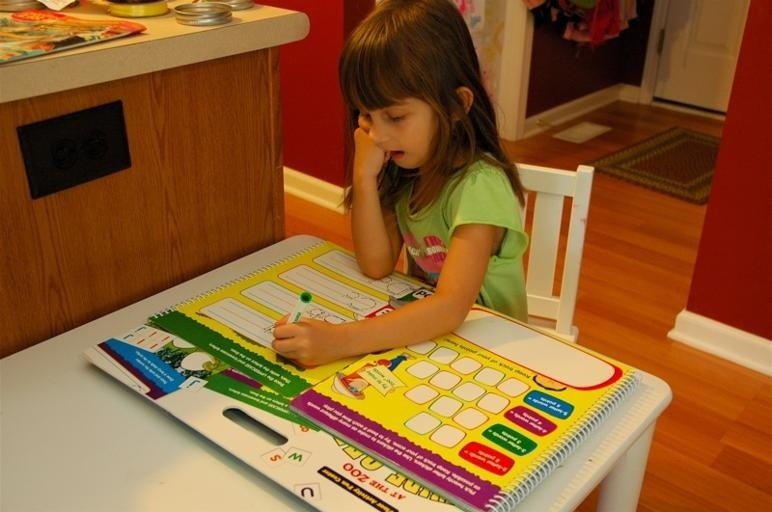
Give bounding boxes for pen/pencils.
[286,291,313,323]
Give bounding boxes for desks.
[0,234,672,512]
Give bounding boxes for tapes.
[110,1,169,18]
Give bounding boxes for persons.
[271,0,529,370]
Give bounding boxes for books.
[147,239,436,399]
[0,10,147,65]
[288,303,644,512]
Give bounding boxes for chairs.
[514,162,595,343]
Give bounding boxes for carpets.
[592,125,722,206]
